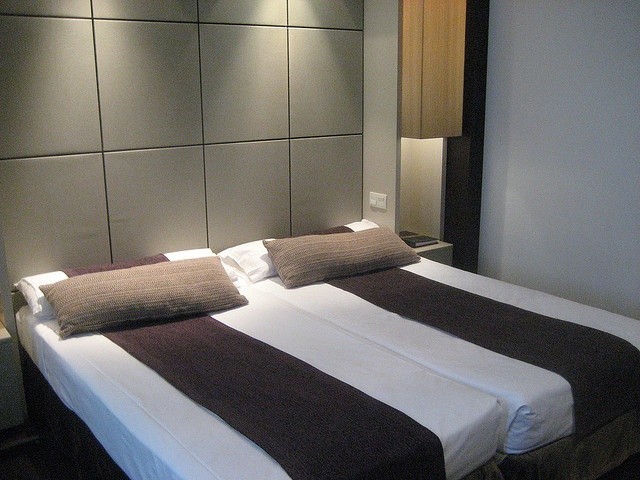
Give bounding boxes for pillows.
[218,218,381,283]
[14,246,242,319]
[261,226,421,289]
[38,256,249,341]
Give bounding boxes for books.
[400,230,439,248]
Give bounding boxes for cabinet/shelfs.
[0,318,29,432]
[412,240,454,267]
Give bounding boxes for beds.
[13,218,640,480]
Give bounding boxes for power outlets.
[369,191,387,210]
